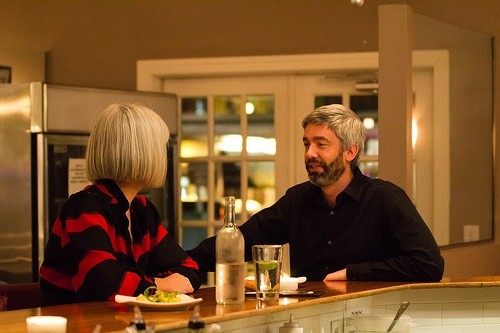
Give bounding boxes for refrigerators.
[0,81,182,285]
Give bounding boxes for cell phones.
[280,291,324,297]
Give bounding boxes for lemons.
[259,260,277,270]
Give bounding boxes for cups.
[26,316,67,333]
[252,245,282,301]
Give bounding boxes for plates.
[115,294,203,310]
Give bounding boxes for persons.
[38,102,202,307]
[185,104,445,283]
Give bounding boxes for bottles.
[215,196,245,305]
